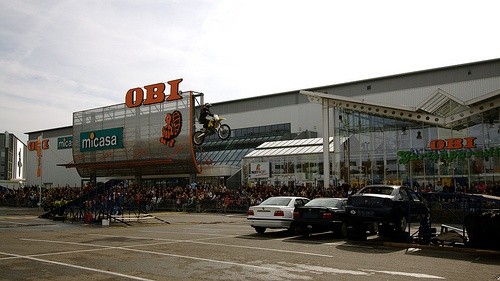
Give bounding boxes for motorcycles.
[192,112,231,145]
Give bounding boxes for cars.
[345,183,431,232]
[293,197,378,238]
[247,196,310,234]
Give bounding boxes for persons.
[198,103,213,129]
[1,175,500,226]
